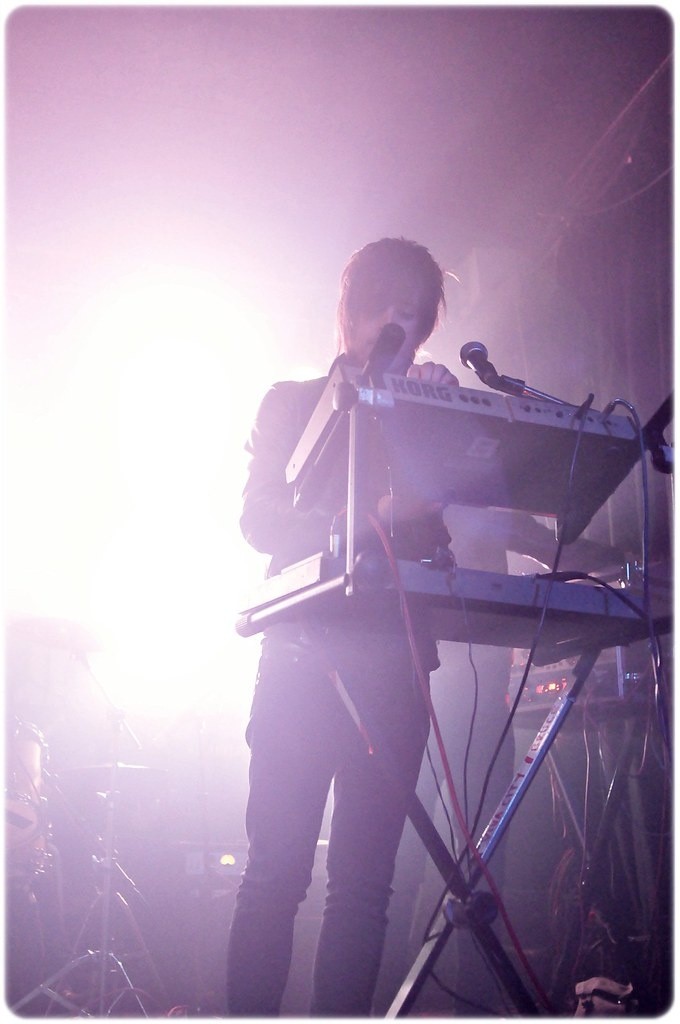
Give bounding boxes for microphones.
[459,341,502,389]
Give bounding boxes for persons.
[223,237,462,1019]
[372,502,636,1019]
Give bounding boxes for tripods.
[9,649,170,1019]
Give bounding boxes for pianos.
[285,364,645,547]
[232,545,675,661]
